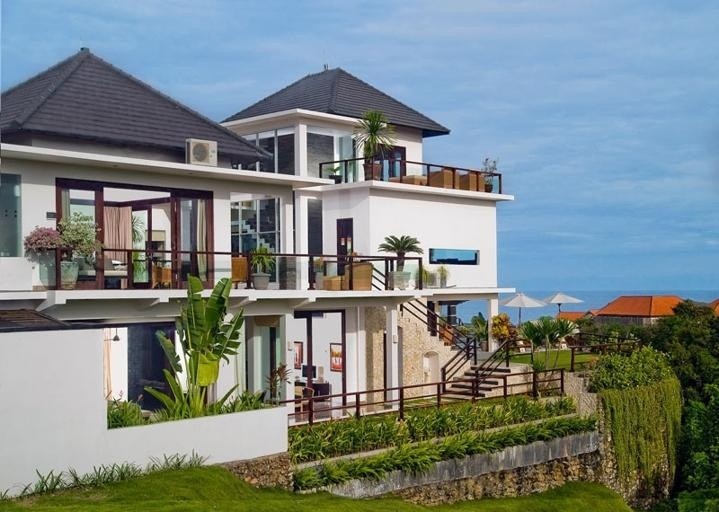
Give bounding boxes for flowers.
[23,225,63,252]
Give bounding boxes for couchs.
[461,172,486,193]
[318,263,373,291]
[429,169,459,190]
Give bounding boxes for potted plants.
[379,234,424,290]
[60,209,108,287]
[352,109,397,181]
[471,313,490,353]
[482,161,496,193]
[325,166,342,184]
[246,247,276,290]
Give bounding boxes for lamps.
[110,328,121,343]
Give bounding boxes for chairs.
[232,255,249,287]
[149,268,171,284]
[294,386,311,412]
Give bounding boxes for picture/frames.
[293,340,302,369]
[328,342,341,371]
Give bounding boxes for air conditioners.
[183,139,218,168]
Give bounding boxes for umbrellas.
[542,291,585,313]
[498,291,546,327]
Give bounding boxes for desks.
[298,380,331,401]
[388,175,425,185]
